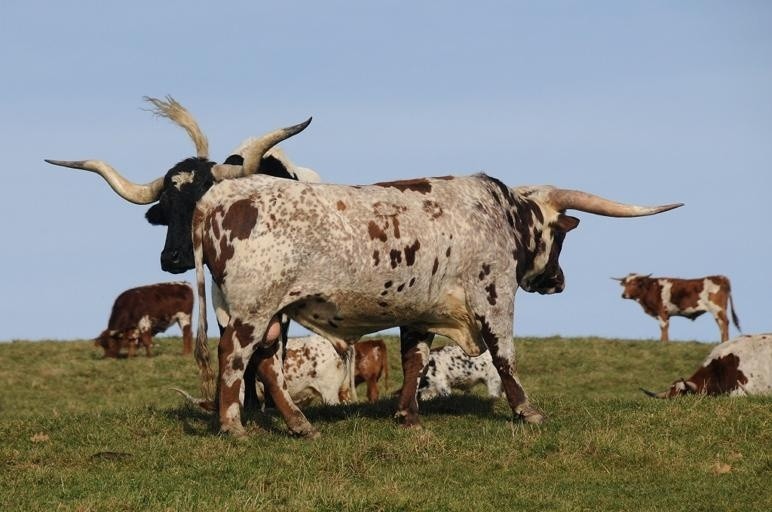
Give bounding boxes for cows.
[190,173,686,445]
[44,95,324,274]
[638,332,772,402]
[610,272,742,343]
[167,334,358,418]
[392,342,506,402]
[93,282,194,358]
[353,338,389,405]
